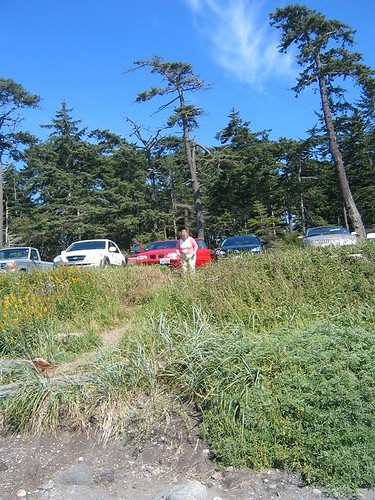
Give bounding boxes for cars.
[52,239,126,268]
[213,235,269,261]
[297,224,360,249]
[135,237,210,268]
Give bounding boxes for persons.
[175,230,199,278]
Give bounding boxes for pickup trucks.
[1,247,54,272]
